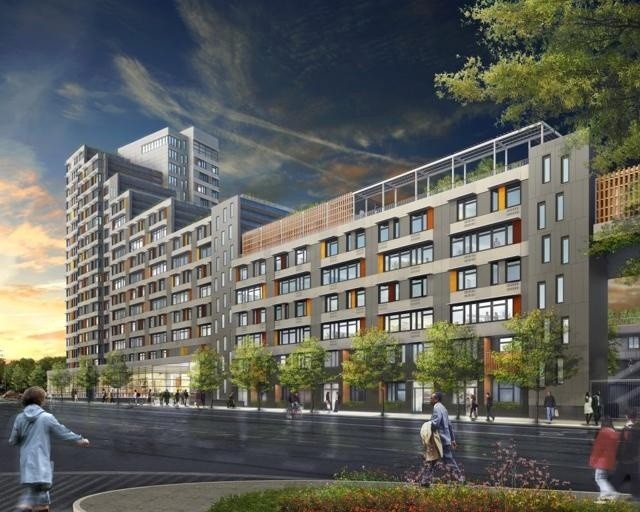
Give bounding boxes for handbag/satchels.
[554,408,559,417]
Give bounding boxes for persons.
[292,392,304,418]
[7,385,90,512]
[485,392,495,421]
[325,391,332,410]
[286,387,295,419]
[543,391,558,424]
[592,390,604,426]
[589,415,621,505]
[615,408,640,501]
[468,394,479,419]
[45,386,236,409]
[334,391,342,411]
[417,391,466,488]
[584,390,594,426]
